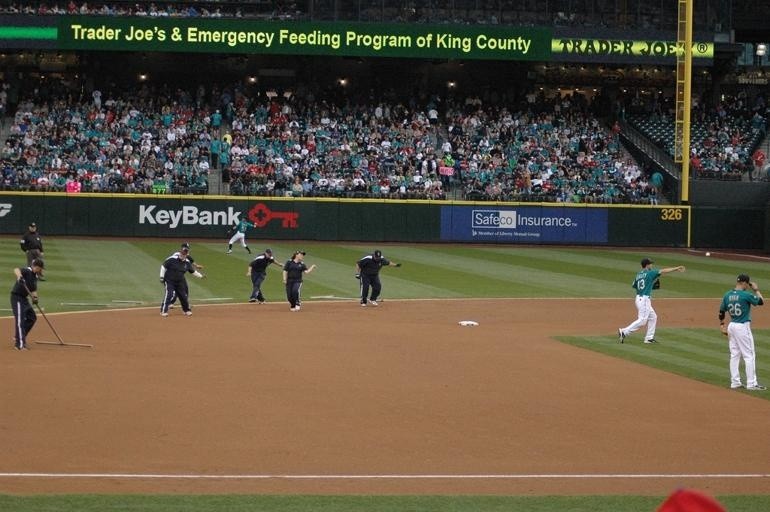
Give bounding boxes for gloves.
[17,276,26,284]
[32,296,38,304]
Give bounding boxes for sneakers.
[160,311,169,317]
[359,300,378,307]
[250,298,266,305]
[617,327,626,342]
[12,335,29,351]
[182,310,192,317]
[735,383,768,391]
[225,249,232,254]
[643,339,658,344]
[289,304,301,313]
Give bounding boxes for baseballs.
[706,252,710,256]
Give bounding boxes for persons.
[21,222,46,281]
[160,247,206,317]
[226,215,257,254]
[10,258,47,351]
[719,274,767,391]
[618,257,685,343]
[247,248,284,303]
[168,243,203,309]
[283,250,318,311]
[356,251,402,307]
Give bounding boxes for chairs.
[1,81,769,207]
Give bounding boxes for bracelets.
[719,323,725,325]
[754,288,759,291]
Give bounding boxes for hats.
[29,221,38,228]
[265,247,272,255]
[640,257,654,267]
[295,250,306,256]
[33,258,47,271]
[375,250,381,258]
[736,274,752,286]
[180,243,190,255]
[241,213,248,218]
